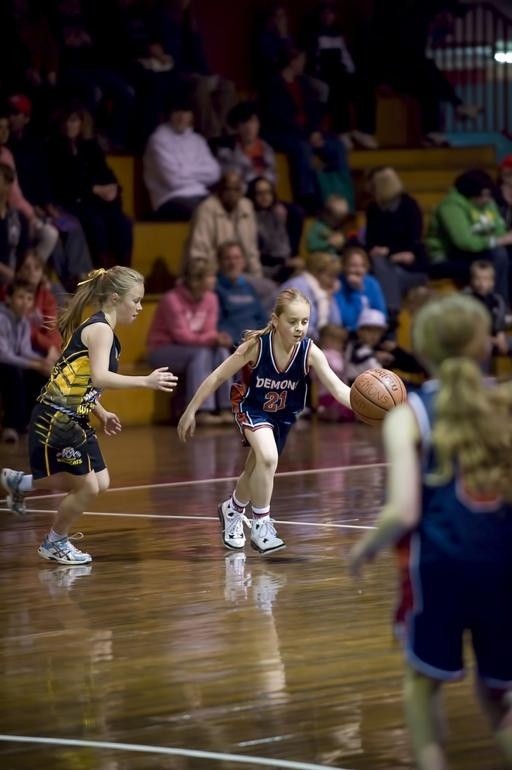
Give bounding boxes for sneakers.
[217,498,251,550]
[0,468,29,515]
[37,531,92,565]
[250,513,286,555]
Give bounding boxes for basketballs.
[349,366,406,424]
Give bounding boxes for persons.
[212,552,306,711]
[345,292,512,769]
[171,289,351,554]
[2,3,511,443]
[2,268,180,564]
[34,567,112,668]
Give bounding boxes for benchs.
[67,144,512,426]
